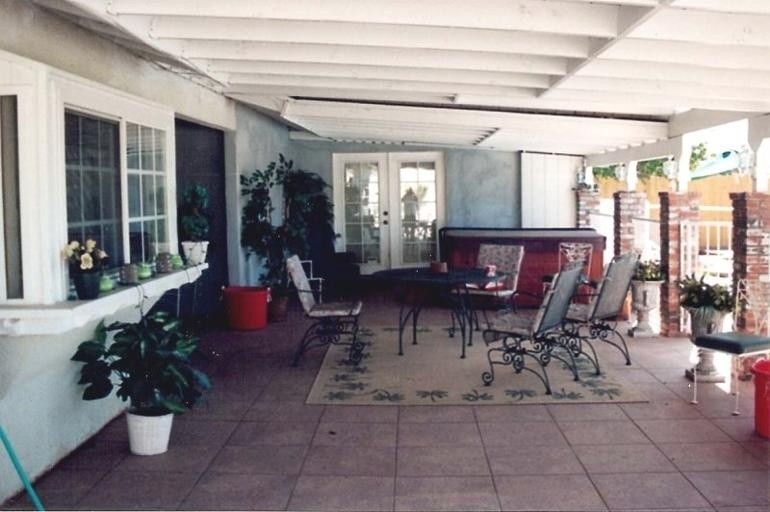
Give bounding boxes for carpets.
[304,325,651,407]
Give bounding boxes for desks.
[373,267,507,358]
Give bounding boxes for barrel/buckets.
[224,283,271,331]
[750,356,766,435]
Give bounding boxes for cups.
[485,265,496,277]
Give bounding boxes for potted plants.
[70,310,216,457]
[673,272,748,383]
[181,183,210,266]
[239,153,335,323]
[628,259,666,338]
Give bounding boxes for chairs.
[286,255,363,368]
[690,279,770,415]
[565,253,641,374]
[448,243,524,337]
[481,269,579,395]
[543,242,595,295]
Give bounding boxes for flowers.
[61,239,109,269]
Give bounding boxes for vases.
[73,271,101,300]
